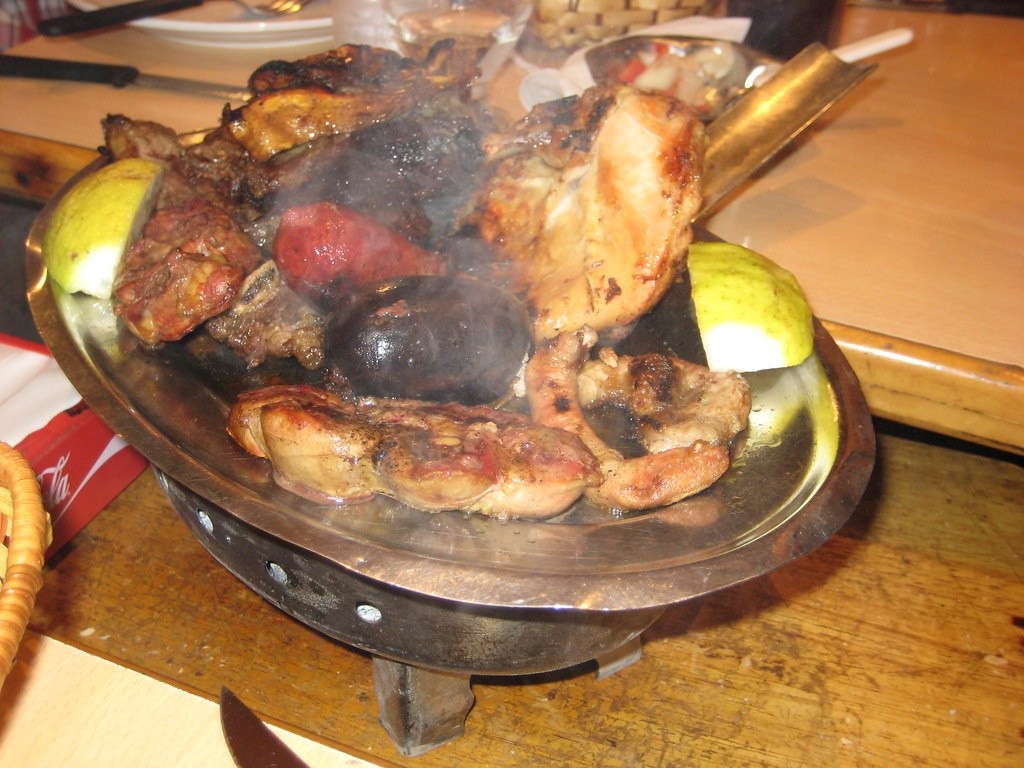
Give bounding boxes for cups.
[382,0,535,104]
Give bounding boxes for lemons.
[686,240,814,374]
[40,156,167,300]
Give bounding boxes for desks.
[0,355,1024,768]
[0,0,1024,456]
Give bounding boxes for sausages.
[274,205,531,405]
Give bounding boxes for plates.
[67,0,334,49]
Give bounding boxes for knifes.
[0,53,254,102]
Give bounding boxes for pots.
[25,128,876,756]
